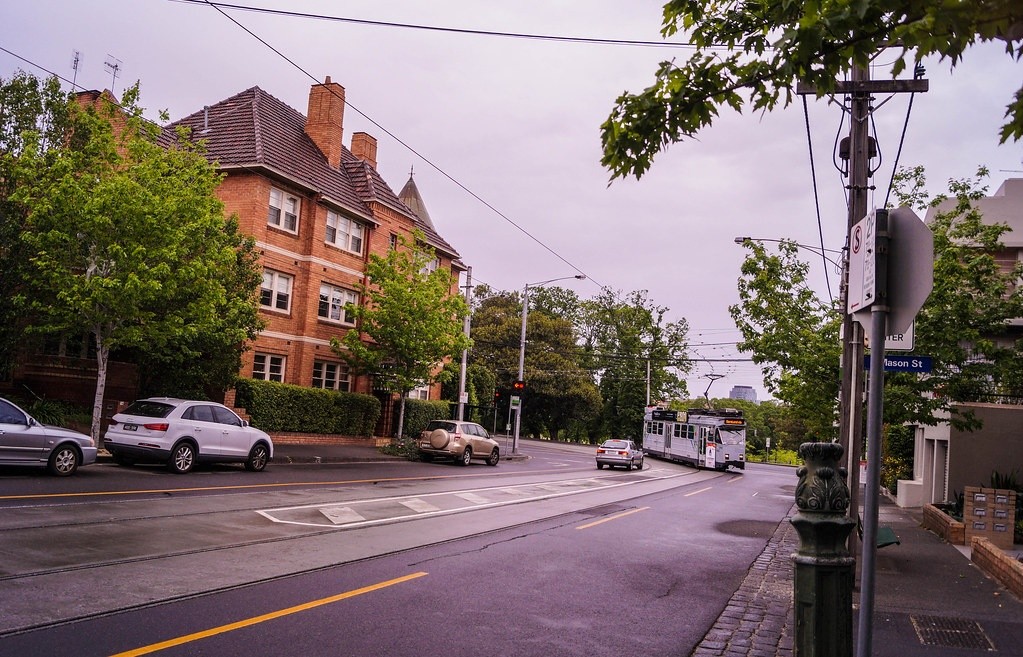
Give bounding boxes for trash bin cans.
[100,399,129,451]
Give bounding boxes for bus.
[643,405,747,472]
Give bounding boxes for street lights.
[512,275,586,453]
[734,236,845,472]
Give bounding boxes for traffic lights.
[494,387,499,403]
[513,381,526,390]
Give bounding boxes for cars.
[0,397,97,477]
[596,439,643,471]
[104,397,273,474]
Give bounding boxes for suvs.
[420,420,500,466]
